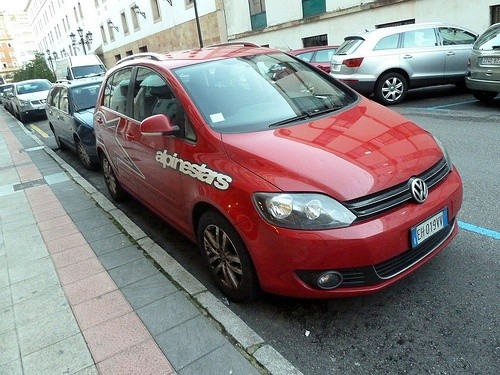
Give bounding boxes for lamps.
[133,4,146,19]
[69,33,77,42]
[107,20,118,31]
[86,31,92,40]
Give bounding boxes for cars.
[272,21,480,105]
[0,75,112,171]
[466,23,500,103]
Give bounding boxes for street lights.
[40,49,57,81]
[69,26,93,55]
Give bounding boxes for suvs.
[94,41,463,305]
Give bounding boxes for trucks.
[52,53,108,80]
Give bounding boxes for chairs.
[208,67,259,109]
[77,89,94,107]
[19,87,25,94]
